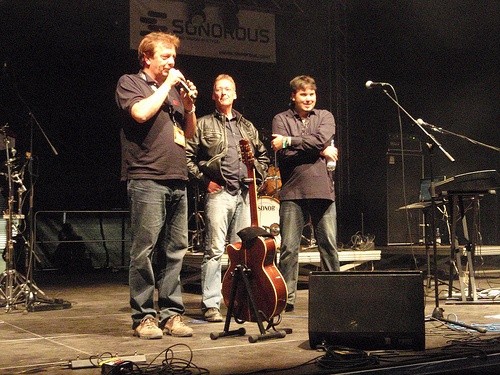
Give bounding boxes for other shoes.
[284,303,294,312]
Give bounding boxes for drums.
[261,165,282,197]
[247,196,281,252]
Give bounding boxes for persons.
[115,30,198,340]
[186,74,270,322]
[271,75,339,312]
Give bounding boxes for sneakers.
[134,319,163,340]
[160,313,193,337]
[203,307,223,322]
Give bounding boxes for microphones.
[365,80,391,90]
[415,118,442,131]
[167,67,196,98]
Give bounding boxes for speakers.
[363,153,425,246]
[308,270,425,351]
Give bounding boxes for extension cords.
[69,354,146,369]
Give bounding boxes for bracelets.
[283,136,289,148]
[184,103,196,115]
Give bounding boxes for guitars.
[222,139,289,323]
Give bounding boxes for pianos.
[422,169,500,305]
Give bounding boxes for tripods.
[188,177,205,253]
[0,141,47,311]
[424,125,487,333]
